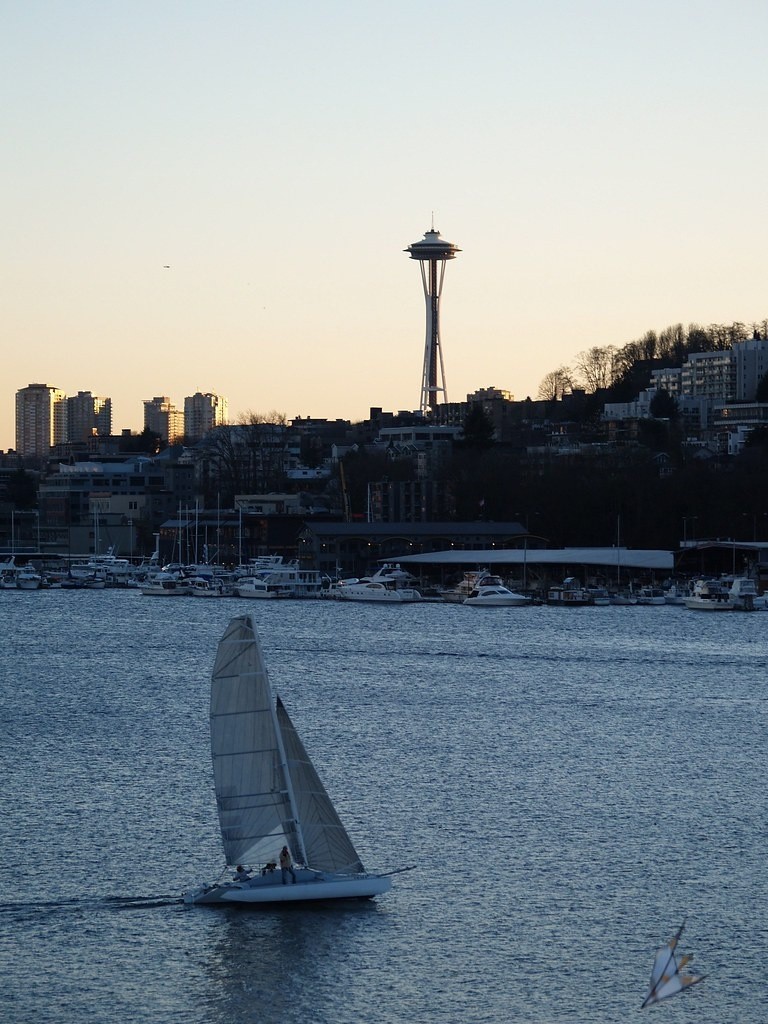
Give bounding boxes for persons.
[260,860,277,869]
[232,865,253,882]
[279,846,297,885]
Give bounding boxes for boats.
[0,492,768,611]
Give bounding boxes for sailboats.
[181,611,394,908]
